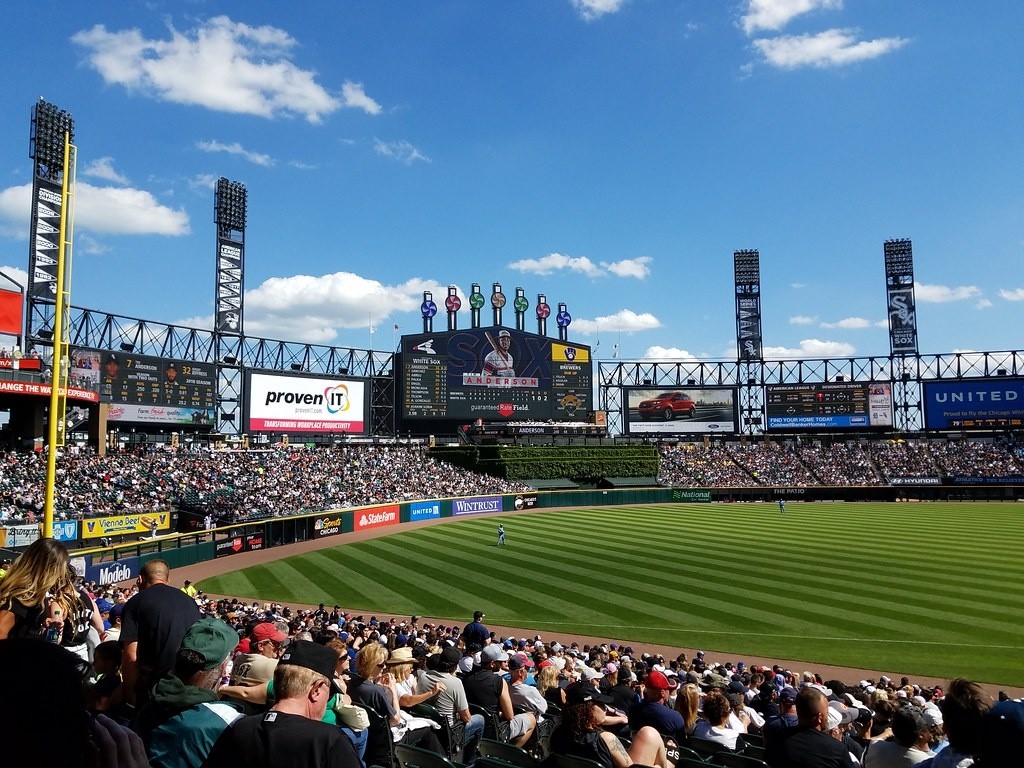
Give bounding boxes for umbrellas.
[896,439,905,443]
[886,439,895,444]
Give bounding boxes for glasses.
[376,663,385,669]
[339,652,349,661]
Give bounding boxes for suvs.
[637,391,696,422]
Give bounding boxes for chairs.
[348,699,771,768]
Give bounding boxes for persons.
[1,345,51,384]
[658,437,1024,486]
[165,363,179,385]
[778,499,787,514]
[72,350,101,370]
[482,329,516,377]
[68,373,98,391]
[497,524,507,547]
[105,354,122,380]
[0,443,533,540]
[0,537,1024,768]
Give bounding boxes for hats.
[970,701,1024,768]
[197,591,944,731]
[459,656,473,673]
[177,618,240,670]
[508,654,534,671]
[249,623,289,643]
[481,645,510,663]
[473,652,483,666]
[386,647,418,664]
[277,640,344,695]
[644,670,677,689]
[538,661,553,671]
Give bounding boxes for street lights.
[216,177,246,435]
[27,96,77,360]
[885,236,924,431]
[734,245,766,437]
